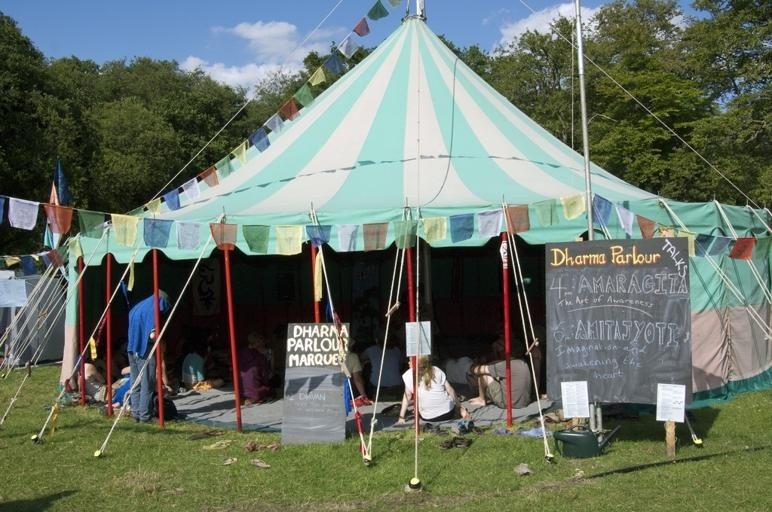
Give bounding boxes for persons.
[127,281,174,424]
[77,336,531,425]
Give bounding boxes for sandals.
[440,436,472,450]
[422,422,449,437]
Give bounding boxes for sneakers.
[450,418,484,436]
[133,418,155,424]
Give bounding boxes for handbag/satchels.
[384,406,411,417]
[553,429,602,458]
[156,399,178,420]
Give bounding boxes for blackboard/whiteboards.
[545,237,693,405]
[281,323,350,445]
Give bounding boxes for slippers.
[492,427,527,436]
